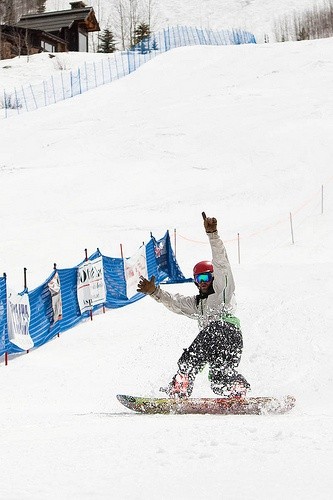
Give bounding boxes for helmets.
[193,260,214,277]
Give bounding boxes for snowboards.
[116,393,297,417]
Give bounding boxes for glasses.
[196,272,212,284]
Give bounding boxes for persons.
[136,210,250,401]
